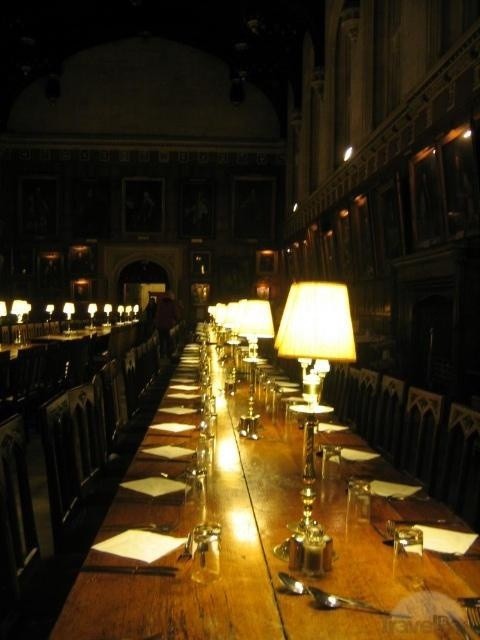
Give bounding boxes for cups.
[344,482,374,534]
[191,321,319,470]
[392,529,428,589]
[185,468,206,505]
[319,444,343,483]
[192,522,226,587]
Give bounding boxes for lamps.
[208,281,358,413]
[0,299,140,320]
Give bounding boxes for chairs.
[0,316,478,638]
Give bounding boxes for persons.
[143,291,185,356]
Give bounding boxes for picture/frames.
[231,175,276,245]
[179,178,214,240]
[70,175,111,240]
[121,174,165,241]
[18,174,60,241]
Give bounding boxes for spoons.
[277,570,412,616]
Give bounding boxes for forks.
[464,606,480,640]
